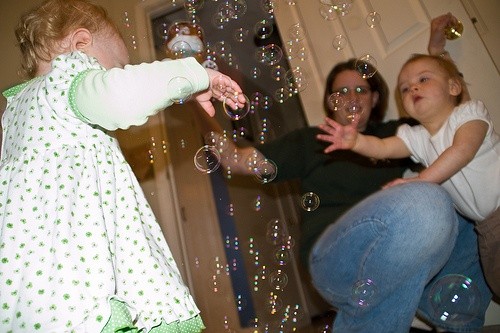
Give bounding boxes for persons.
[175,12,494,332]
[1,0,246,333]
[317,54,499,300]
